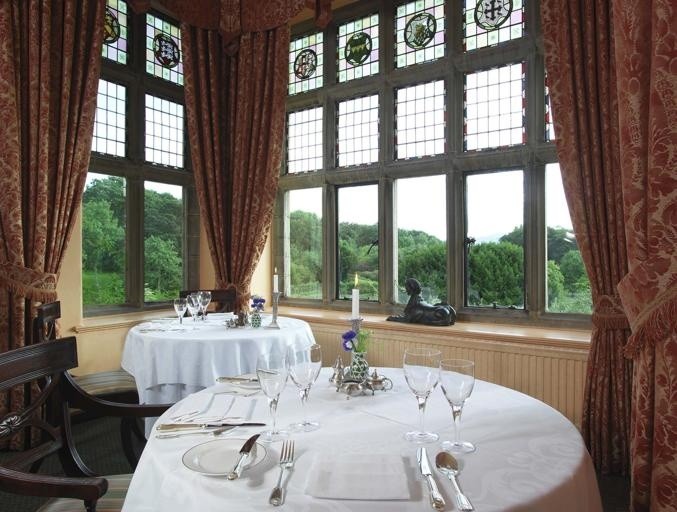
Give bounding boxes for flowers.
[251,295,265,314]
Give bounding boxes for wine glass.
[438,358,477,455]
[286,343,322,432]
[255,353,287,441]
[401,345,440,445]
[174,290,212,331]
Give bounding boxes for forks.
[267,438,296,507]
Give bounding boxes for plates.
[180,436,267,477]
[231,373,261,390]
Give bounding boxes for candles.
[273,266,279,292]
[352,270,360,318]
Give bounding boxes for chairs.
[1,300,179,511]
[180,290,236,317]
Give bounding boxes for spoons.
[434,452,473,512]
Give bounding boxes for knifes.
[225,433,259,479]
[156,419,265,431]
[413,447,445,512]
